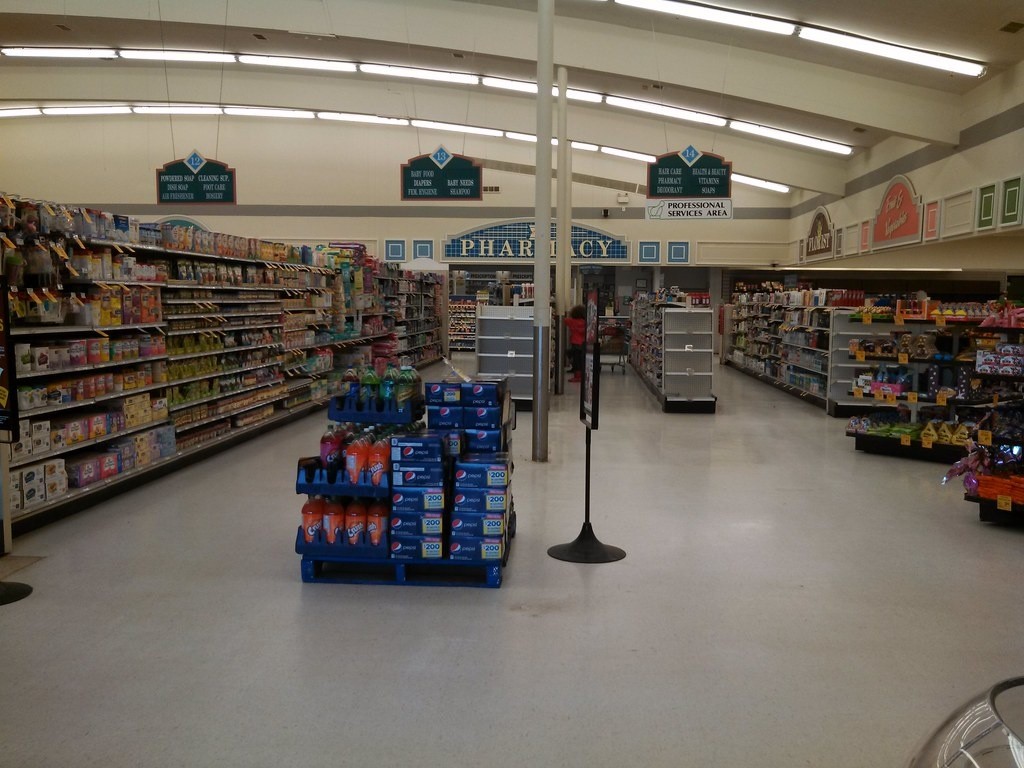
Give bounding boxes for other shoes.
[567,368,575,373]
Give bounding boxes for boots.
[568,370,581,382]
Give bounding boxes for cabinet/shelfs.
[1,215,452,546]
[626,292,717,414]
[719,298,941,419]
[449,293,499,352]
[846,303,1024,448]
[487,278,534,307]
[473,305,557,401]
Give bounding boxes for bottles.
[340,355,421,413]
[321,421,426,484]
[302,495,389,545]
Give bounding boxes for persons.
[564,305,586,382]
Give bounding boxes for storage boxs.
[0,191,1024,563]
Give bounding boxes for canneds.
[15,333,166,410]
